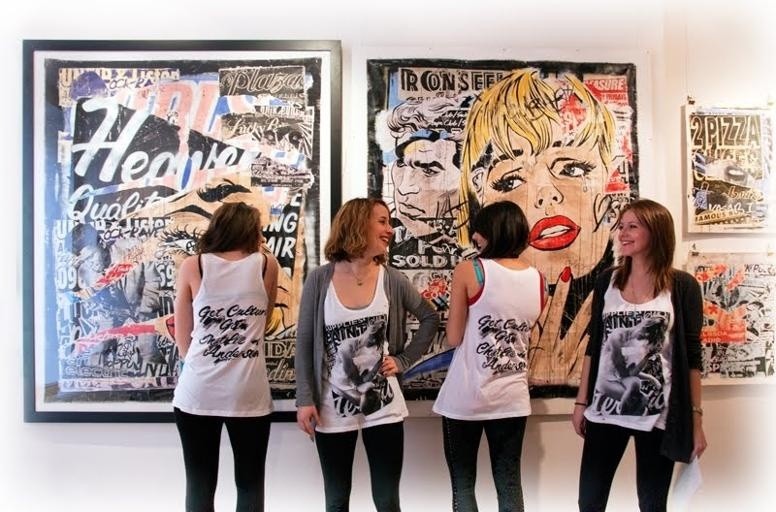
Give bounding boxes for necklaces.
[346,260,372,286]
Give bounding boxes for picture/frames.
[682,101,774,385]
[351,43,659,426]
[21,36,344,425]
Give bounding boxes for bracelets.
[691,406,703,416]
[575,403,587,406]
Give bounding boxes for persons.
[385,94,470,240]
[571,197,709,512]
[432,199,547,512]
[454,65,618,387]
[293,197,440,512]
[169,201,279,512]
